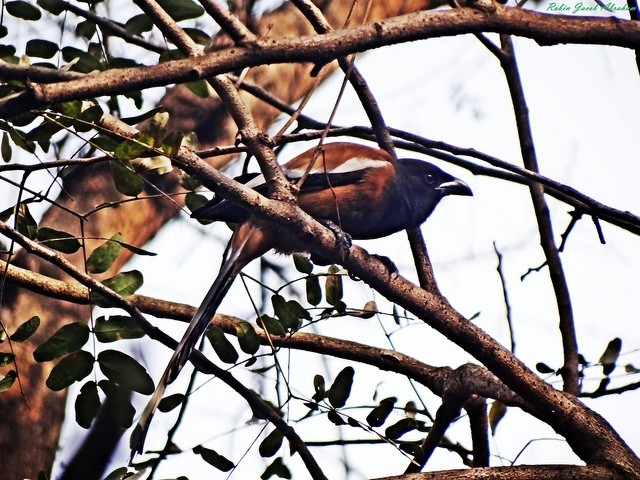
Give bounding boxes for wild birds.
[188,165,374,223]
[124,141,474,467]
